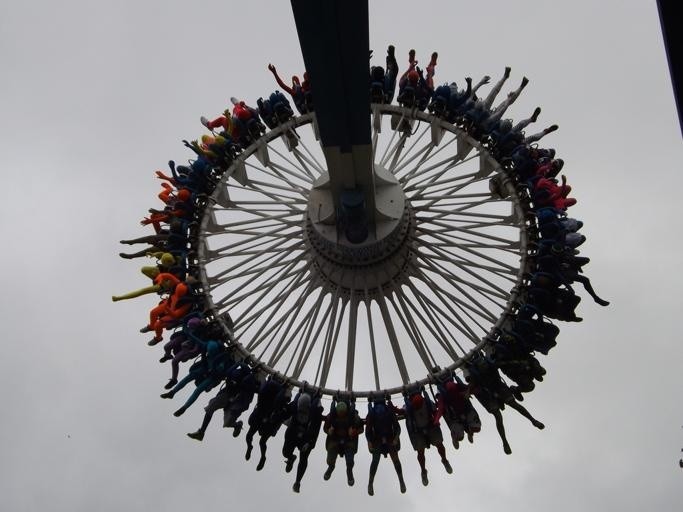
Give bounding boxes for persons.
[109,46,610,495]
